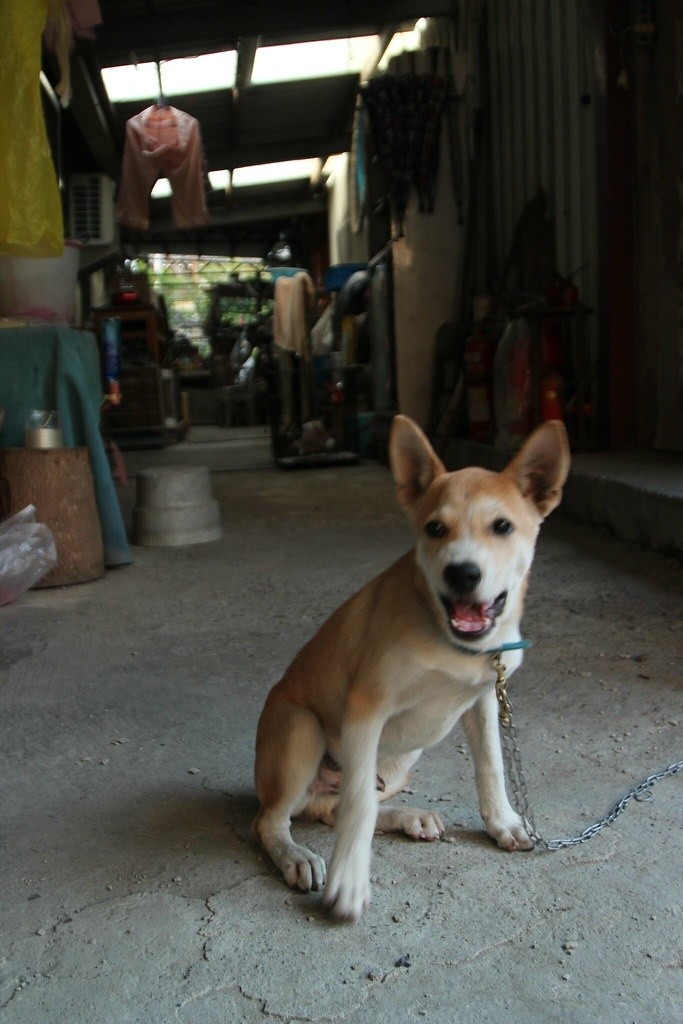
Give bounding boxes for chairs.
[222,346,262,426]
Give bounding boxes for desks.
[92,305,175,420]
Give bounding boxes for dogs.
[252,413,569,923]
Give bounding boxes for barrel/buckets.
[127,465,220,547]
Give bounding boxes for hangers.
[148,94,176,126]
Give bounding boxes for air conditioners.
[68,173,120,247]
